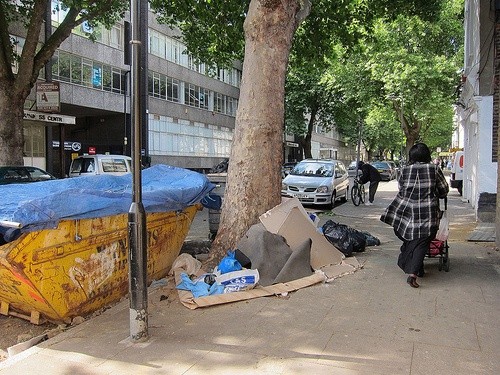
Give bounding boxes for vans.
[68,154,131,178]
[446,151,464,196]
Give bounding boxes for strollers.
[423,195,450,272]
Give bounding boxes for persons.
[358,161,382,206]
[433,158,440,166]
[380,142,449,288]
[441,159,444,171]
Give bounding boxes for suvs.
[281,158,349,210]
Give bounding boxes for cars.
[0,164,56,185]
[348,161,357,170]
[368,160,406,182]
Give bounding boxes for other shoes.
[365,201,373,205]
[407,276,419,288]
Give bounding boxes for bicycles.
[350,174,367,206]
